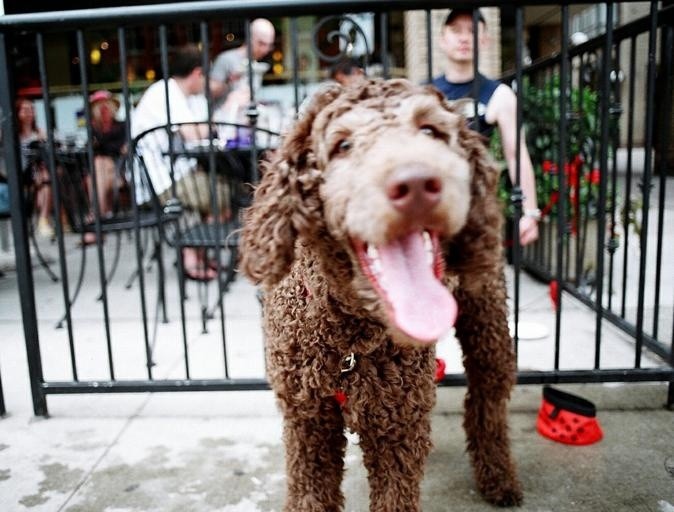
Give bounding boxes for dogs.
[225,65,525,512]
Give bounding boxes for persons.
[82,90,131,245]
[210,18,275,121]
[421,7,540,247]
[15,98,56,242]
[131,46,241,214]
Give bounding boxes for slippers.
[174,262,217,282]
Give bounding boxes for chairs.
[21,117,287,367]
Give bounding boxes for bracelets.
[524,208,542,222]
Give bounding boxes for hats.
[445,8,486,26]
